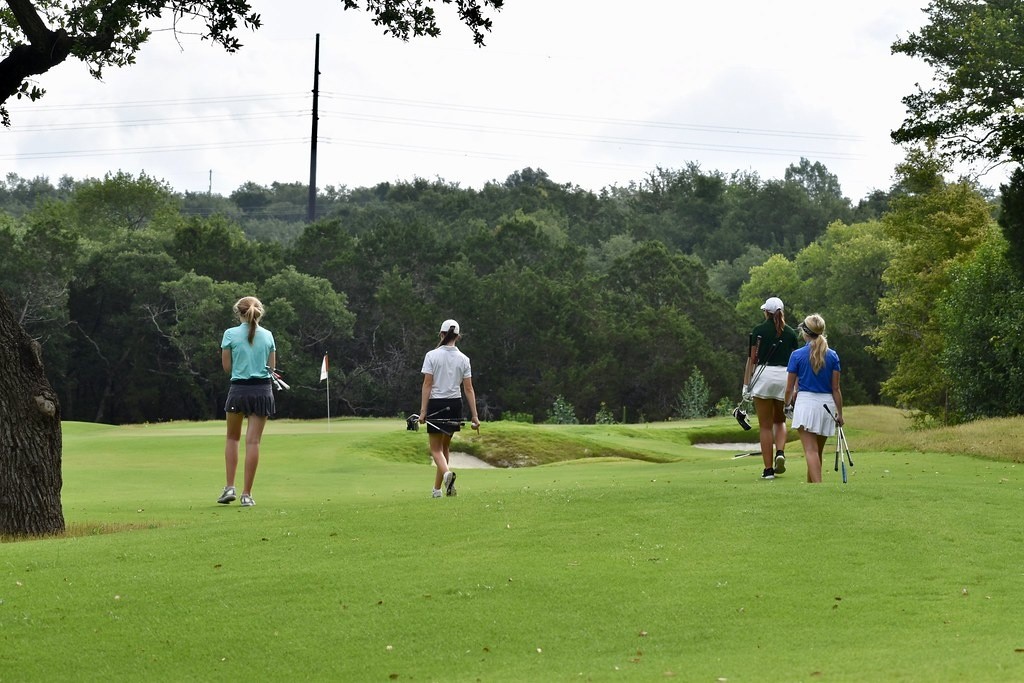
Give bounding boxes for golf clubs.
[823,405,854,484]
[404,407,469,440]
[266,361,291,393]
[733,332,785,432]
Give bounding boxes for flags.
[319,354,328,383]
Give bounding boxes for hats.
[760,297,784,314]
[441,319,460,334]
[798,320,819,338]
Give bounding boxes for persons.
[783,314,846,483]
[215,297,276,507]
[418,319,479,497]
[742,295,798,479]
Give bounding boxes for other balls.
[472,422,477,427]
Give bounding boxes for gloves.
[742,384,751,403]
[783,403,793,419]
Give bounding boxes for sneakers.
[774,450,786,474]
[240,494,255,506]
[217,487,237,502]
[761,468,775,479]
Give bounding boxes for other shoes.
[444,472,457,497]
[432,490,442,498]
